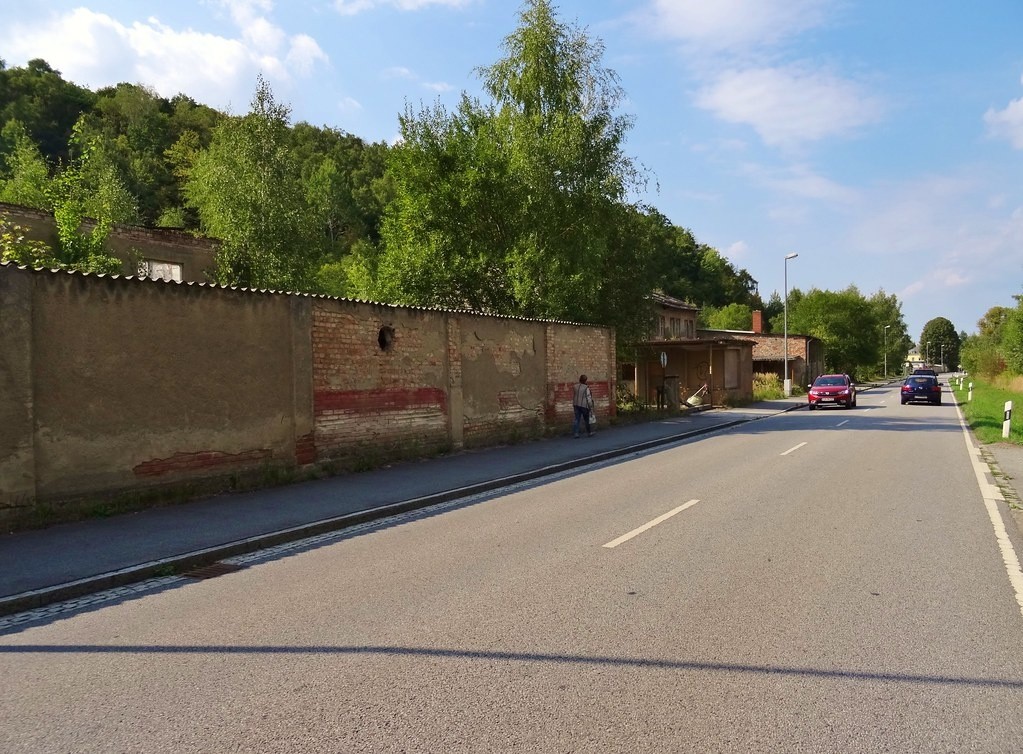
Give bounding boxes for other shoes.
[575,433,579,438]
[588,432,596,437]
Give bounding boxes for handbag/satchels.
[589,412,596,424]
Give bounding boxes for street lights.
[927,342,931,364]
[884,325,891,377]
[783,252,798,396]
[941,345,944,373]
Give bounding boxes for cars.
[900,375,943,404]
[808,373,856,409]
[910,370,938,383]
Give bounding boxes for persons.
[572,374,597,438]
